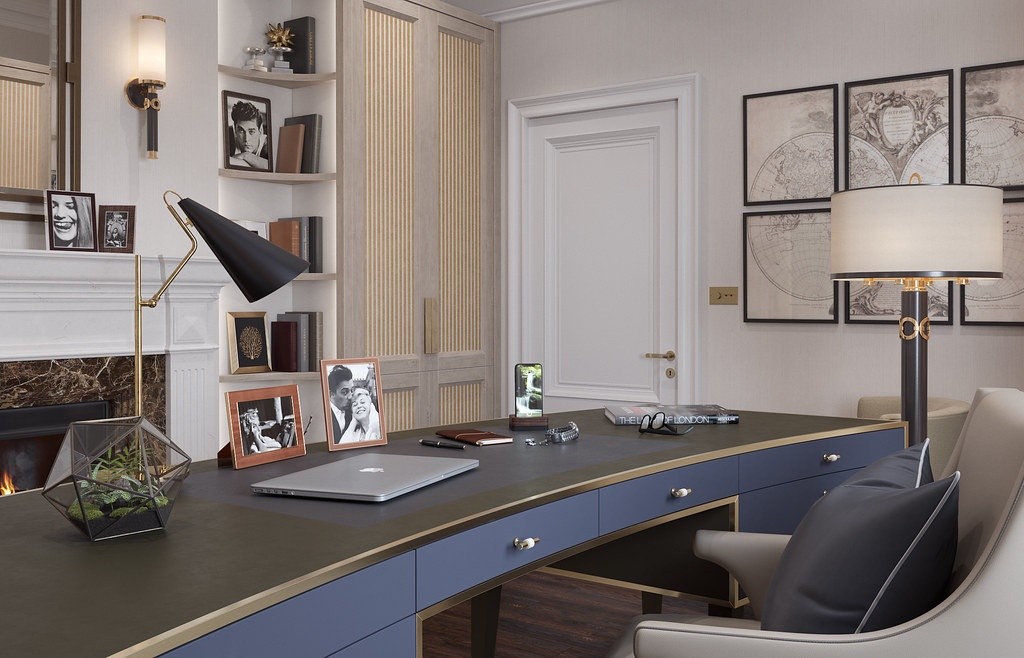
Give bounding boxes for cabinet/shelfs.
[217,1,339,380]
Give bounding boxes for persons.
[326,365,381,445]
[239,408,283,456]
[108,227,126,247]
[51,194,92,247]
[226,101,268,170]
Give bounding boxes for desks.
[0,407,909,658]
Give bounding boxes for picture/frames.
[218,384,306,470]
[227,312,274,374]
[43,189,98,254]
[743,59,1024,328]
[319,359,387,454]
[234,220,268,241]
[222,89,273,174]
[97,204,136,253]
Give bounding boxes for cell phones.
[514,363,544,417]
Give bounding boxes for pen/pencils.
[419,438,466,450]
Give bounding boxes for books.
[604,405,741,426]
[283,15,316,74]
[435,429,513,447]
[268,214,324,273]
[271,307,324,372]
[274,113,324,173]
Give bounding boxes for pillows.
[761,438,961,633]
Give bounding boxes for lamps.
[85,191,311,486]
[831,182,1004,444]
[125,15,167,159]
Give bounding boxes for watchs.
[545,421,579,443]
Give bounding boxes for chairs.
[607,386,1024,657]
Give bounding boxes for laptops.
[251,453,480,502]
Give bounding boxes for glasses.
[639,412,694,435]
